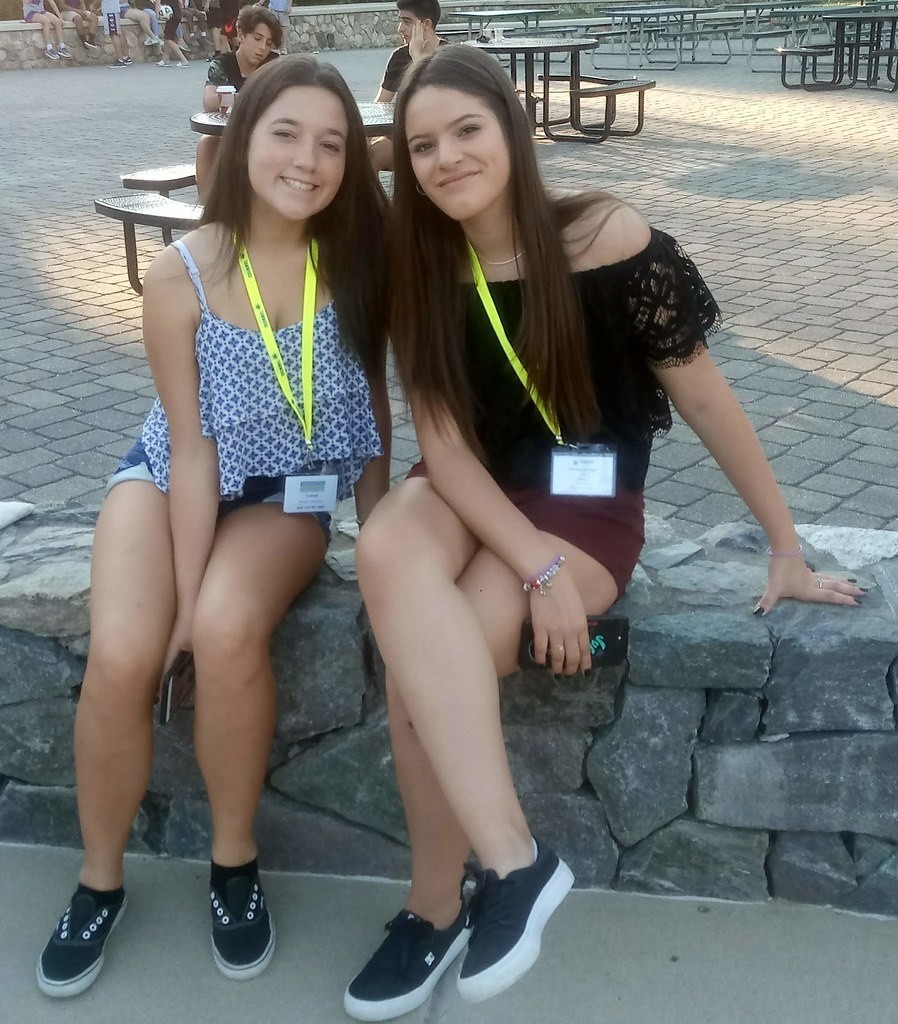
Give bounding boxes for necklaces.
[479,250,524,265]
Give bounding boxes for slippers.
[156,60,172,67]
[176,61,191,68]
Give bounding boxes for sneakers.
[144,35,157,45]
[37,876,115,999]
[208,870,274,984]
[153,36,164,46]
[84,41,101,50]
[453,834,574,1006]
[177,38,192,52]
[122,56,132,65]
[341,904,460,1020]
[57,47,72,59]
[45,50,59,60]
[107,59,127,68]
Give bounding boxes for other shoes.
[271,48,288,54]
[201,31,207,37]
[206,55,217,62]
[190,32,195,38]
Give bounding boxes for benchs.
[96,163,205,297]
[435,7,898,93]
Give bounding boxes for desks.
[769,5,881,91]
[458,38,599,139]
[190,101,396,136]
[448,11,557,41]
[716,0,807,56]
[604,8,716,67]
[592,5,689,51]
[865,1,898,12]
[823,10,898,89]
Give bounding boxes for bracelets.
[356,520,365,524]
[767,544,802,557]
[522,553,565,596]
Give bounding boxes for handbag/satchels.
[220,18,238,39]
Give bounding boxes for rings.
[551,645,563,651]
[819,581,823,588]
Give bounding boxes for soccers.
[157,5,173,22]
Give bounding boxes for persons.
[196,5,283,206]
[369,0,451,175]
[150,0,190,67]
[58,0,98,50]
[37,54,393,998]
[201,0,239,62]
[345,44,868,1022]
[90,0,132,68]
[178,0,206,37]
[257,0,292,54]
[128,0,190,51]
[119,0,164,45]
[22,0,72,59]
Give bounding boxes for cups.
[482,29,492,40]
[494,28,503,42]
[216,86,236,114]
